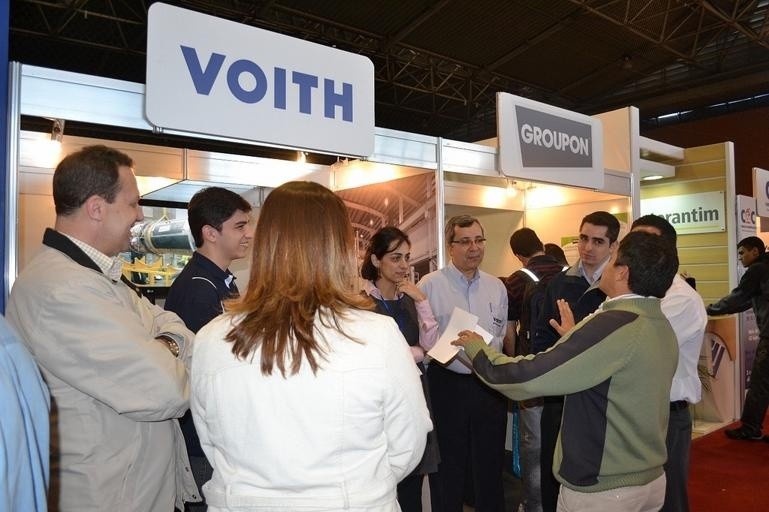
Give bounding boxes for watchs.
[157,333,181,357]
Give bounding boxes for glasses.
[451,238,487,250]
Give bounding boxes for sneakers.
[724,424,764,440]
[519,499,531,511]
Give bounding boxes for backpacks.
[520,266,570,355]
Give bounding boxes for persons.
[706,235,769,444]
[187,179,438,509]
[4,145,205,512]
[161,186,255,512]
[0,310,52,511]
[414,212,513,510]
[351,223,440,509]
[503,211,710,511]
[448,230,682,510]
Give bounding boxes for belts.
[670,401,688,412]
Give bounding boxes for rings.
[460,335,469,340]
